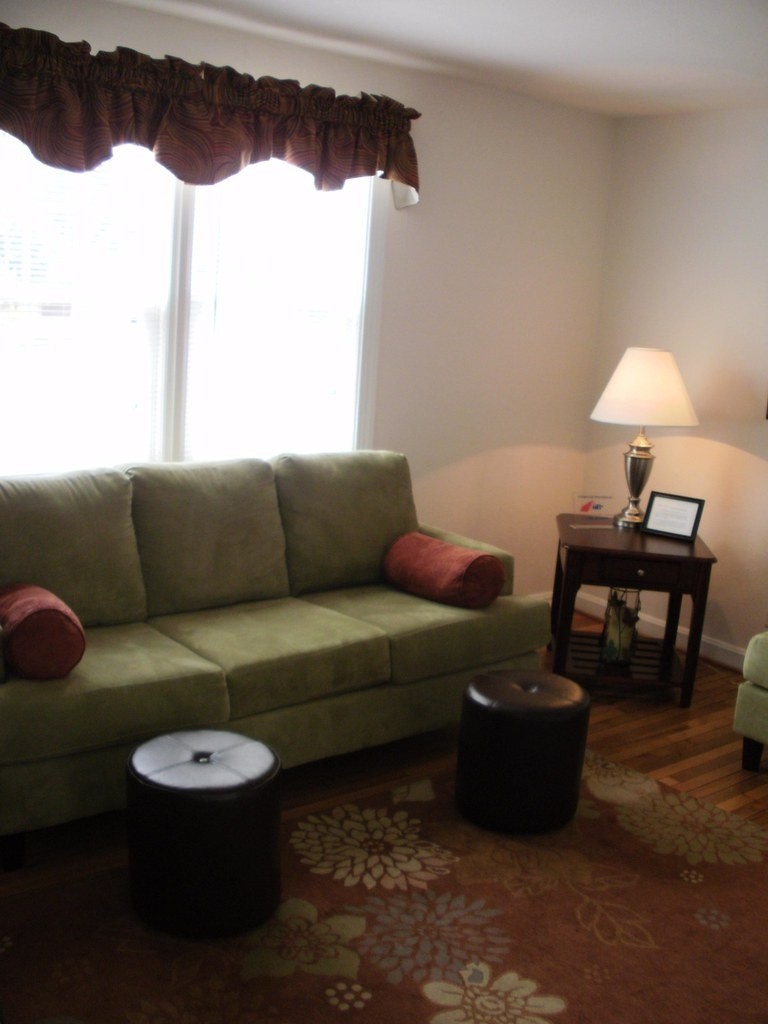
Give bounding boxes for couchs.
[0,450,554,838]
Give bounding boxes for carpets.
[0,735,768,1024]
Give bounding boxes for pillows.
[383,532,505,610]
[0,582,86,678]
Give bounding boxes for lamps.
[589,348,699,529]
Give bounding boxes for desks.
[548,513,717,707]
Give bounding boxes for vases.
[597,587,641,666]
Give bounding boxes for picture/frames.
[640,491,705,543]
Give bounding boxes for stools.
[456,669,589,835]
[126,729,283,936]
[734,629,767,773]
